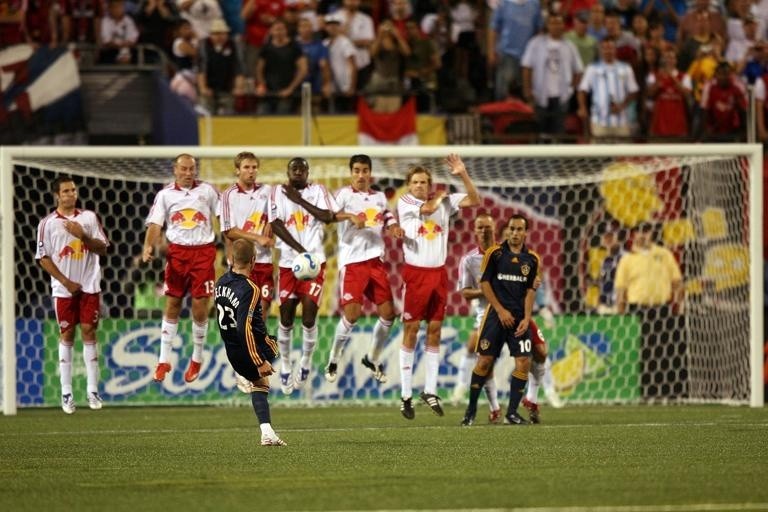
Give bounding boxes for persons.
[325,153,405,386]
[616,221,685,399]
[577,218,627,313]
[213,239,288,446]
[219,152,275,394]
[268,158,334,394]
[1,1,768,141]
[34,177,111,415]
[397,152,480,420]
[143,154,220,383]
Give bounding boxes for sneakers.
[503,412,525,424]
[419,391,444,417]
[61,393,75,413]
[235,373,251,393]
[280,370,294,395]
[153,362,171,382]
[295,363,309,390]
[399,395,414,420]
[361,354,387,382]
[261,432,286,446]
[490,408,501,424]
[461,410,476,427]
[522,395,540,424]
[86,390,103,409]
[323,360,338,383]
[185,359,201,382]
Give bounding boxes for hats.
[209,20,230,34]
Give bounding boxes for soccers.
[292,253,321,281]
